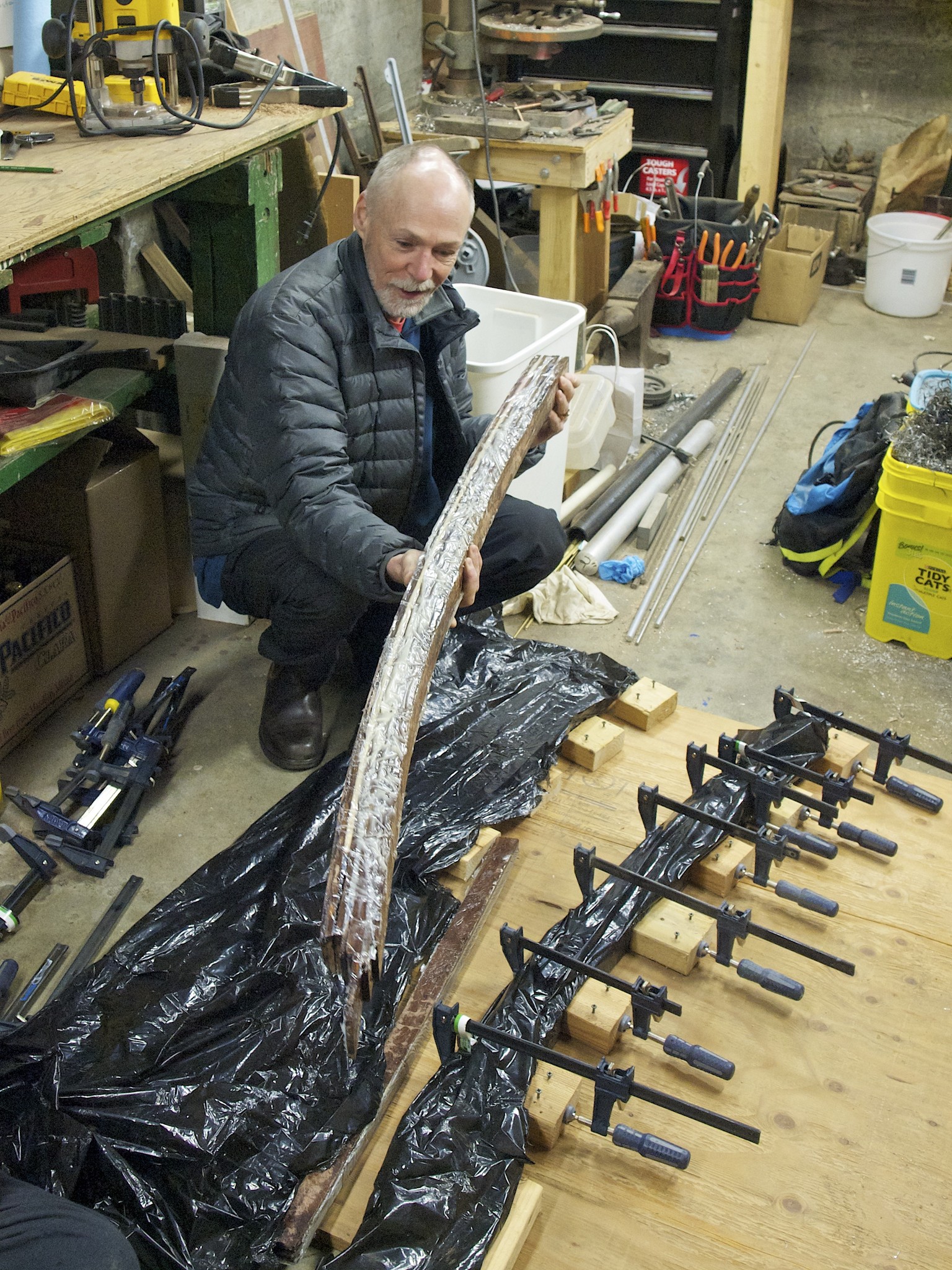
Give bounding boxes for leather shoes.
[259,663,324,770]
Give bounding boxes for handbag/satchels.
[581,326,644,475]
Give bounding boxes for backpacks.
[776,392,914,581]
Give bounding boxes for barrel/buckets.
[860,213,952,319]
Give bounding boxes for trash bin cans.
[864,213,951,318]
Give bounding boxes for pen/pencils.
[1,164,63,173]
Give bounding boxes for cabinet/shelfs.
[0,76,355,624]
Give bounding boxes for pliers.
[719,239,748,269]
[698,230,720,268]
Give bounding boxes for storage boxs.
[104,75,166,104]
[0,418,173,676]
[749,224,835,326]
[611,190,660,225]
[2,71,87,119]
[0,537,90,764]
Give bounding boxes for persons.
[189,143,581,772]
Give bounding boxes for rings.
[556,409,570,420]
